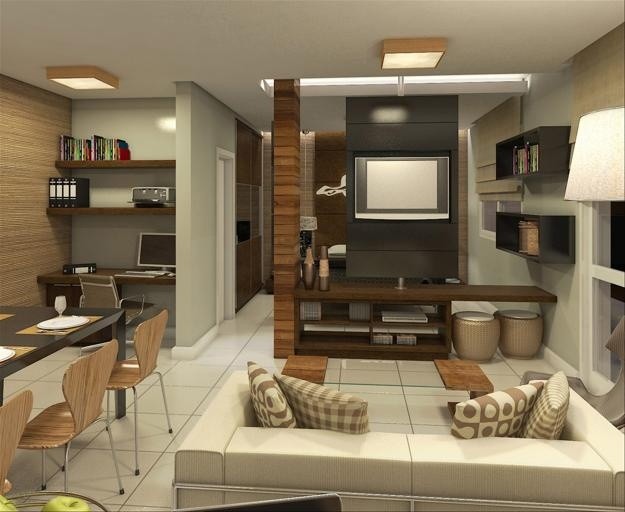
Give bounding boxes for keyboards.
[115,270,163,278]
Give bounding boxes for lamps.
[46,66,119,90]
[381,38,448,70]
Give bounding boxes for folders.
[49,178,89,208]
[61,263,97,274]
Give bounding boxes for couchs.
[179,371,625,512]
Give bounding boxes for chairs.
[78,274,145,357]
[0,305,173,498]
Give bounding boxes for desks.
[37,262,176,305]
[294,267,558,302]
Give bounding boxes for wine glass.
[55,296,67,318]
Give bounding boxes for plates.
[37,316,89,330]
[0,348,16,363]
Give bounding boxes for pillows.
[451,381,544,439]
[246,361,299,427]
[521,371,570,440]
[274,372,373,435]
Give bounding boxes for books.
[348,302,370,320]
[372,334,418,345]
[511,144,538,175]
[56,134,127,160]
[299,302,322,321]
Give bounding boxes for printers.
[127,187,175,208]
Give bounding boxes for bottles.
[319,246,329,291]
[303,247,316,290]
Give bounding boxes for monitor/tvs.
[138,232,176,272]
[354,156,450,221]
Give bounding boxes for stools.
[451,311,500,362]
[493,310,544,360]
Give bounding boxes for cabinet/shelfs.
[45,160,176,216]
[495,212,575,265]
[294,297,452,361]
[496,125,571,180]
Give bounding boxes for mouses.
[167,273,175,277]
